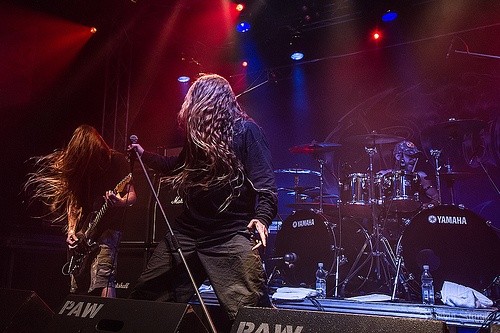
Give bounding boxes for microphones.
[445,40,454,60]
[469,155,476,165]
[129,135,138,153]
[268,70,279,82]
[285,253,297,262]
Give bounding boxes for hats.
[393,141,419,159]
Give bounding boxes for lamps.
[288,22,304,61]
[176,66,190,83]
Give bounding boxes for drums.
[269,207,375,295]
[338,172,375,216]
[373,168,425,214]
[394,201,497,299]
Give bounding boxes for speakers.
[0,288,450,333]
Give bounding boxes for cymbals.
[346,132,406,147]
[286,142,344,154]
[421,120,489,142]
[275,167,323,180]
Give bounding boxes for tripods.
[340,154,412,303]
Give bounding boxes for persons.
[394,142,439,209]
[128,74,279,321]
[25,125,136,297]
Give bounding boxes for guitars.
[66,171,135,283]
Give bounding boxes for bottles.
[316,263,326,299]
[421,264,434,305]
